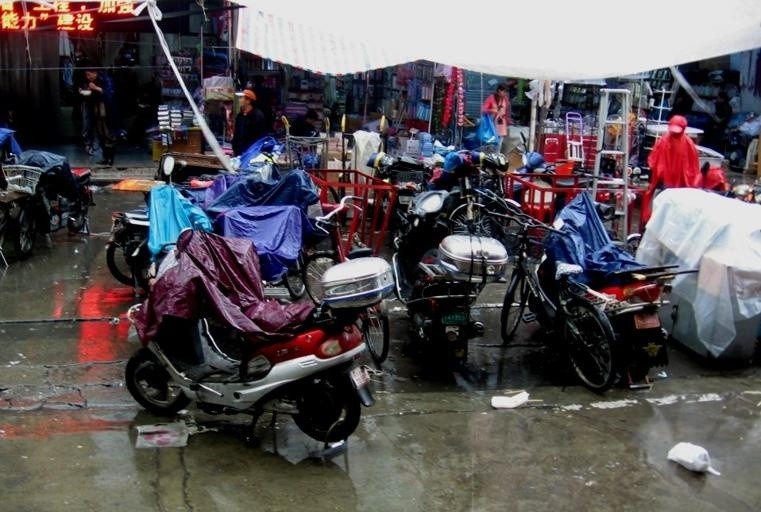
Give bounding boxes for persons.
[229,88,269,157]
[484,82,511,153]
[83,64,115,168]
[638,114,706,236]
[287,108,321,137]
[69,58,96,157]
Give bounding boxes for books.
[155,103,200,131]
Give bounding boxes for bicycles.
[300,194,391,365]
[487,211,618,394]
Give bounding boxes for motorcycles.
[12,150,96,260]
[123,220,396,444]
[108,157,596,291]
[528,190,676,390]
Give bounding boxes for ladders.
[593,89,629,250]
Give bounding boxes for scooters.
[392,189,509,384]
